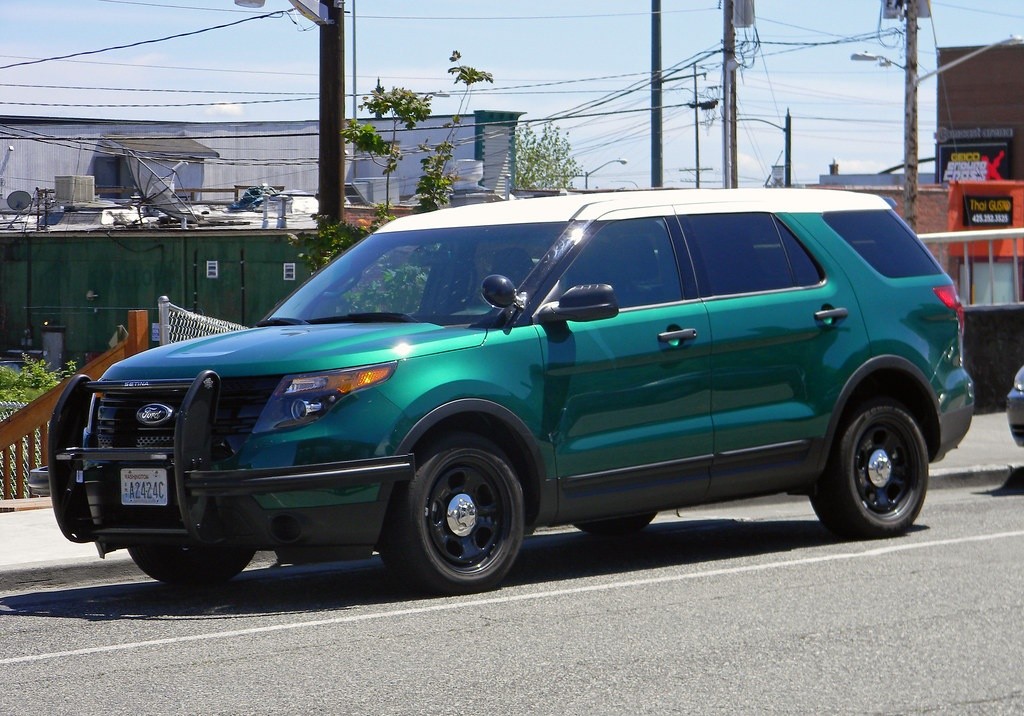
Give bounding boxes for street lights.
[235,0,347,224]
[720,117,792,187]
[850,34,1024,239]
[584,158,627,189]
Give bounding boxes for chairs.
[491,246,536,289]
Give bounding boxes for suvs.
[20,185,979,598]
